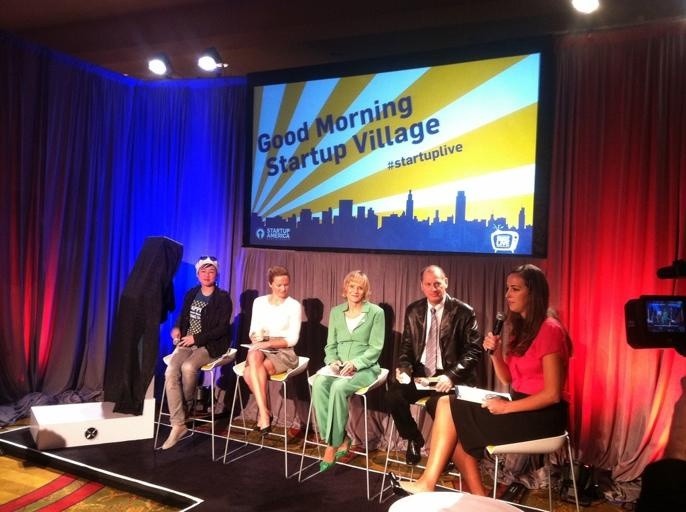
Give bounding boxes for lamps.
[197,46,232,78]
[146,49,177,79]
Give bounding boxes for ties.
[423,308,438,378]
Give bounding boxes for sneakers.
[161,424,188,450]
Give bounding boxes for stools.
[294,364,394,500]
[374,388,465,506]
[485,431,581,511]
[387,491,521,511]
[222,354,322,480]
[149,346,248,461]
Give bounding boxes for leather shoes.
[320,437,352,474]
[405,434,426,465]
[389,471,410,498]
[254,412,275,437]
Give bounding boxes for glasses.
[200,255,217,262]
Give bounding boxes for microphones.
[485,311,507,355]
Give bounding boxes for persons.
[388,264,487,471]
[312,270,386,473]
[392,264,572,496]
[244,264,303,434]
[161,256,232,451]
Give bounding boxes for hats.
[194,257,219,273]
